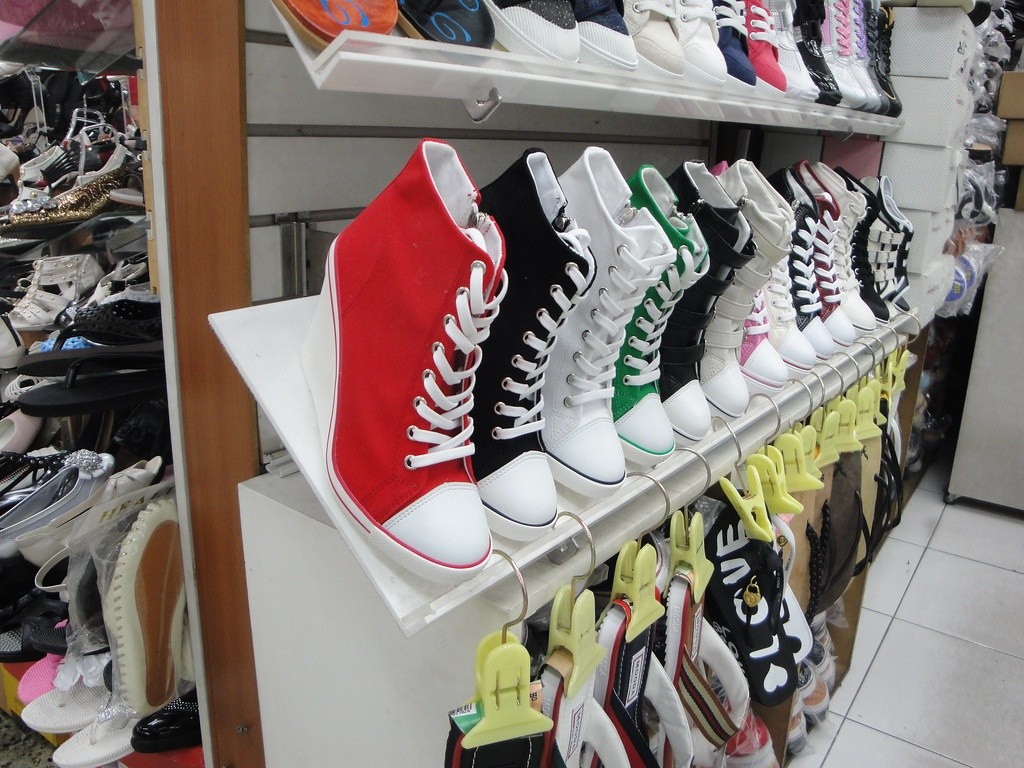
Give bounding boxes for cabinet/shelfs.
[203,0,922,768]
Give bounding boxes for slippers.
[444,348,920,768]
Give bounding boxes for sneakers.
[300,138,915,585]
[279,0,902,117]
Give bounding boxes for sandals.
[0,66,203,768]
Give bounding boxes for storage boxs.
[1002,120,1024,212]
[997,71,1024,119]
